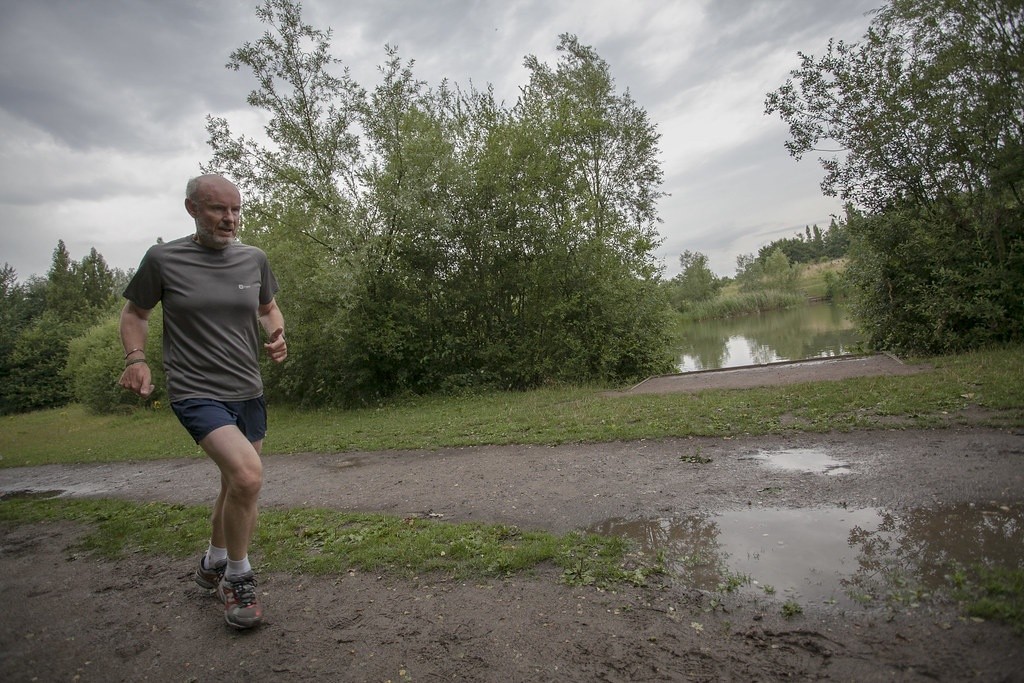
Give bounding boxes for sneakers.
[215,570,262,631]
[194,556,227,589]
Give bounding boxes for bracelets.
[124,349,145,358]
[126,359,147,367]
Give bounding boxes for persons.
[118,175,288,630]
